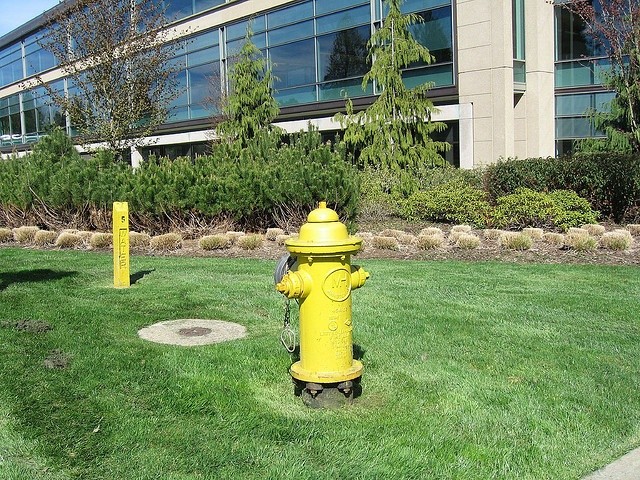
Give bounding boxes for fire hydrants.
[276,202,369,397]
